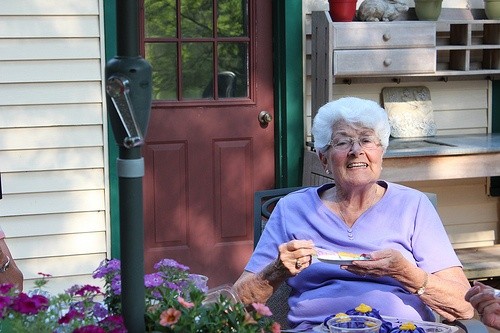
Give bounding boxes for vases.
[484,0,500,19]
[414,0,443,21]
[328,0,357,22]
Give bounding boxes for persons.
[227,96,474,333]
[0,228,24,292]
[464,280,500,331]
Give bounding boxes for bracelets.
[0,255,11,272]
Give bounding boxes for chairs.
[252,187,488,333]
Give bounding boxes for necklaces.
[336,188,376,227]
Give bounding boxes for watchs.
[412,270,429,294]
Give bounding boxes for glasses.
[322,136,382,148]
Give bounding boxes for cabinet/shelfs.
[303,7,500,280]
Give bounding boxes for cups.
[414,0,443,21]
[326,316,382,333]
[328,0,358,22]
[483,0,500,20]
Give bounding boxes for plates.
[312,315,459,333]
[317,253,373,266]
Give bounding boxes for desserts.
[320,302,427,333]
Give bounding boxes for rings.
[295,258,302,268]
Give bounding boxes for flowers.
[0,252,282,333]
